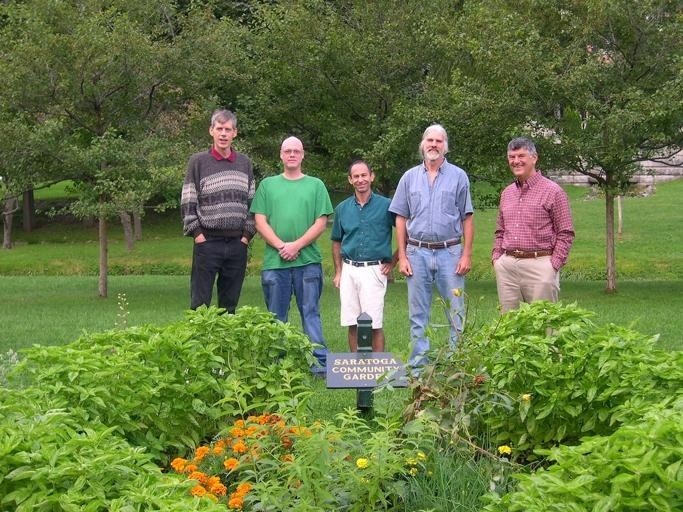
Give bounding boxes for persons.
[249,137,334,378]
[388,124,474,380]
[331,160,409,353]
[490,137,576,363]
[181,109,256,316]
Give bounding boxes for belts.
[407,238,461,249]
[500,250,552,258]
[343,258,380,267]
